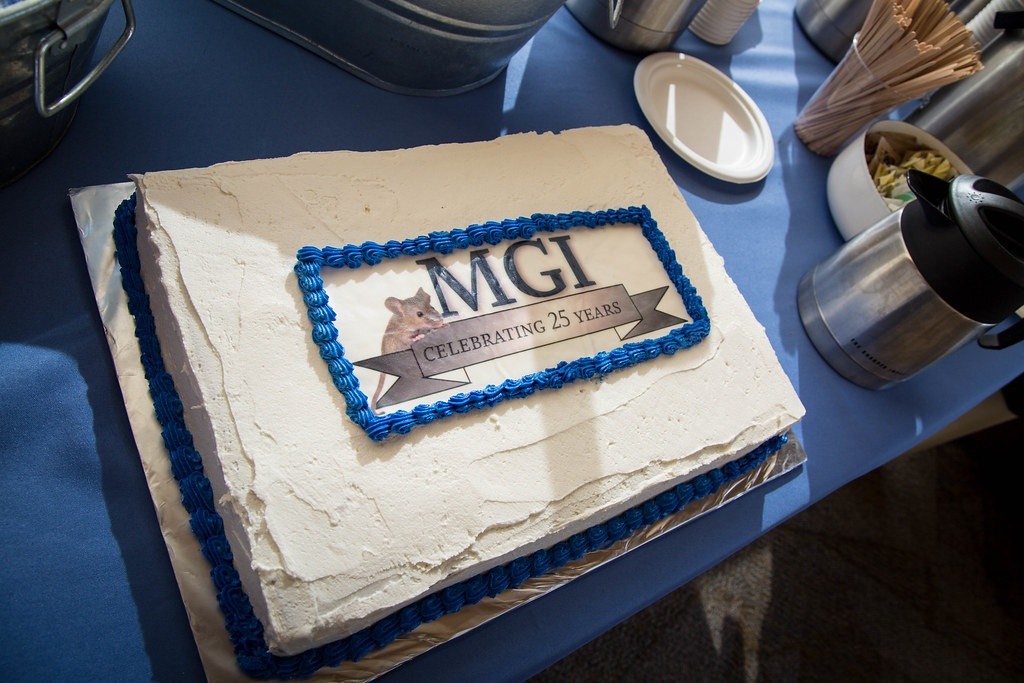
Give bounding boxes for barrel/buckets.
[211,0,565,96]
[564,0,707,52]
[0,0,136,189]
[902,9,1024,186]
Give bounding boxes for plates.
[633,52,774,184]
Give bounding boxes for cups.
[689,0,761,45]
[795,31,926,158]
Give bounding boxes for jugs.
[798,173,1024,391]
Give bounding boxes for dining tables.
[0,0,1024,683]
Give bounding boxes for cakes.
[104,122,808,683]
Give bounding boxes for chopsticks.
[795,0,984,156]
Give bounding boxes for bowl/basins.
[826,119,973,241]
